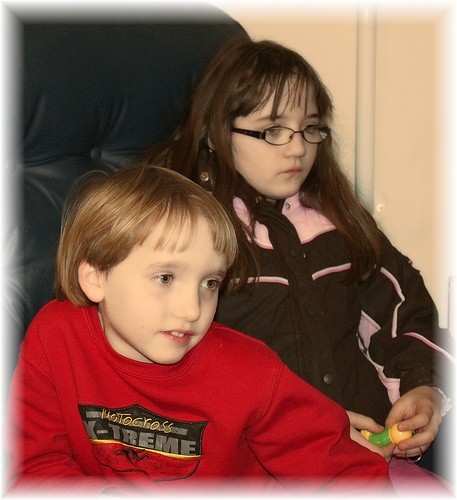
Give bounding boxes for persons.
[4,164,393,493]
[157,41,453,490]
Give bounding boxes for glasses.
[231,124,332,146]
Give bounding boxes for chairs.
[7,8,254,397]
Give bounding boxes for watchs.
[425,383,452,417]
